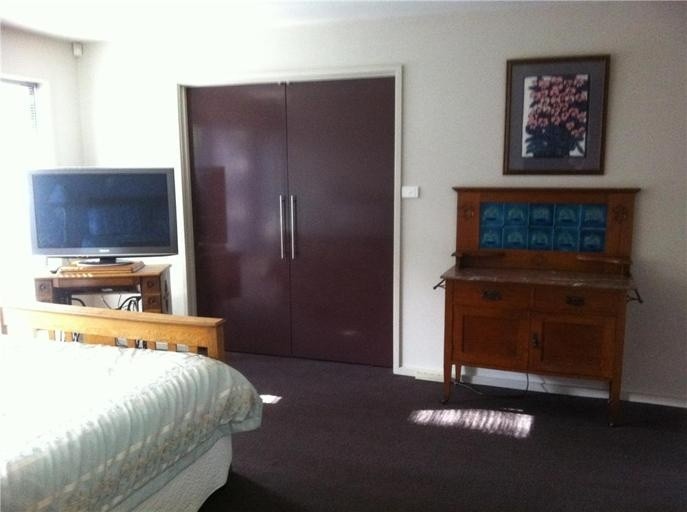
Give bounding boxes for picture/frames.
[502,54,611,175]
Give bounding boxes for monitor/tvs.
[28,166,179,265]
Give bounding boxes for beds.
[0,302,262,512]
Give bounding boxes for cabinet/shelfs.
[441,185,642,417]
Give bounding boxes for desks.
[34,263,174,314]
